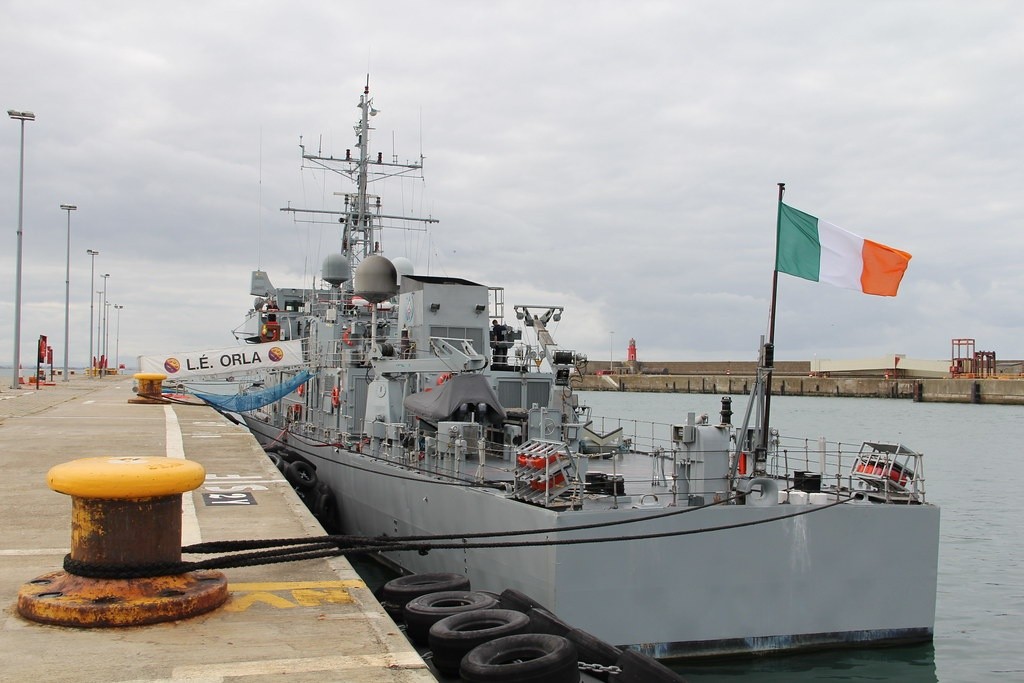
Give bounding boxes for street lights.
[7,110,36,391]
[87,249,99,379]
[114,304,123,368]
[60,205,77,383]
[96,290,103,368]
[101,274,110,377]
[105,301,110,368]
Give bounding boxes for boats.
[235,75,941,666]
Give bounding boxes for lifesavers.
[437,373,452,385]
[331,386,338,408]
[343,328,352,346]
[261,327,277,342]
[298,383,303,396]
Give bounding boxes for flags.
[774,200,912,297]
[47,345,52,364]
[39,334,46,358]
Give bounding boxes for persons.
[267,300,279,311]
[492,319,507,366]
